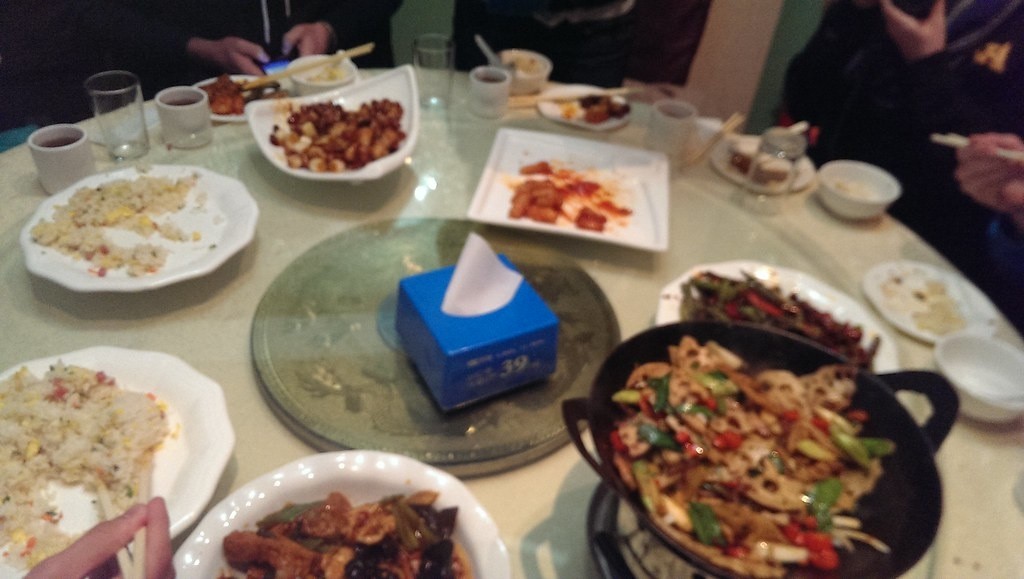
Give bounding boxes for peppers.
[680,269,864,345]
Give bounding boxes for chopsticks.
[238,42,374,92]
[930,131,1024,163]
[506,85,646,109]
[92,449,153,578]
[681,110,747,176]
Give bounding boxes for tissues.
[394,229,559,417]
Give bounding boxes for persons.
[449,0,642,92]
[132,0,403,98]
[21,496,177,579]
[781,0,1024,334]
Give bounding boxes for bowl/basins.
[489,47,553,95]
[817,158,902,219]
[285,54,358,93]
[931,329,1023,424]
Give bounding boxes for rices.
[0,360,169,569]
[29,173,208,277]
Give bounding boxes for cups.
[26,122,99,195]
[470,67,513,118]
[155,86,212,149]
[642,99,699,155]
[83,70,151,161]
[411,33,453,106]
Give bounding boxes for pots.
[556,320,963,578]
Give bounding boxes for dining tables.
[0,65,1024,578]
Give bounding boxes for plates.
[171,448,511,578]
[538,84,633,131]
[18,163,259,293]
[243,63,419,182]
[0,344,237,578]
[707,131,815,193]
[467,125,670,251]
[190,74,293,122]
[863,258,1003,346]
[655,261,899,375]
